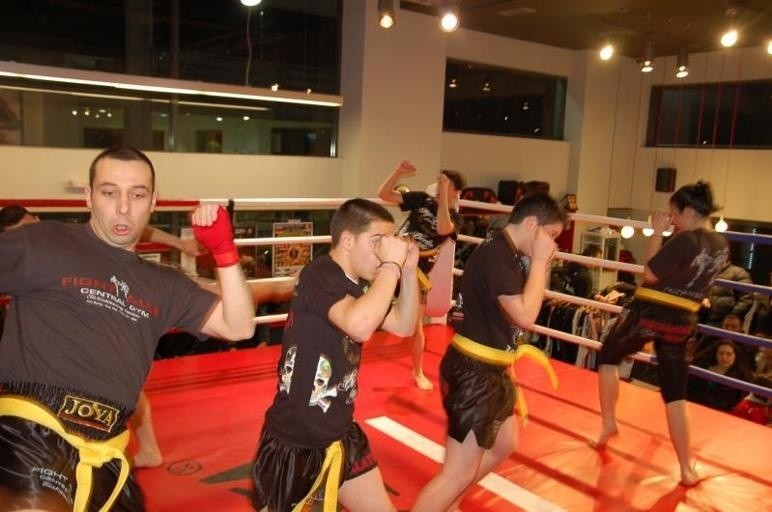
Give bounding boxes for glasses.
[639,41,654,73]
[642,215,654,237]
[720,14,740,50]
[597,35,615,61]
[714,214,728,233]
[621,216,634,239]
[437,1,461,34]
[377,0,396,31]
[677,47,689,79]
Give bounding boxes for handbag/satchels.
[379,259,402,278]
[651,233,665,241]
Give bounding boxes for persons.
[412,192,565,512]
[547,305,600,368]
[702,259,755,327]
[2,203,206,466]
[688,340,753,413]
[0,145,256,511]
[556,244,602,303]
[250,198,423,512]
[380,160,467,392]
[588,179,728,487]
[698,315,756,376]
[730,375,772,427]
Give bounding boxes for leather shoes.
[191,205,239,269]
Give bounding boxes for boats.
[655,167,677,193]
